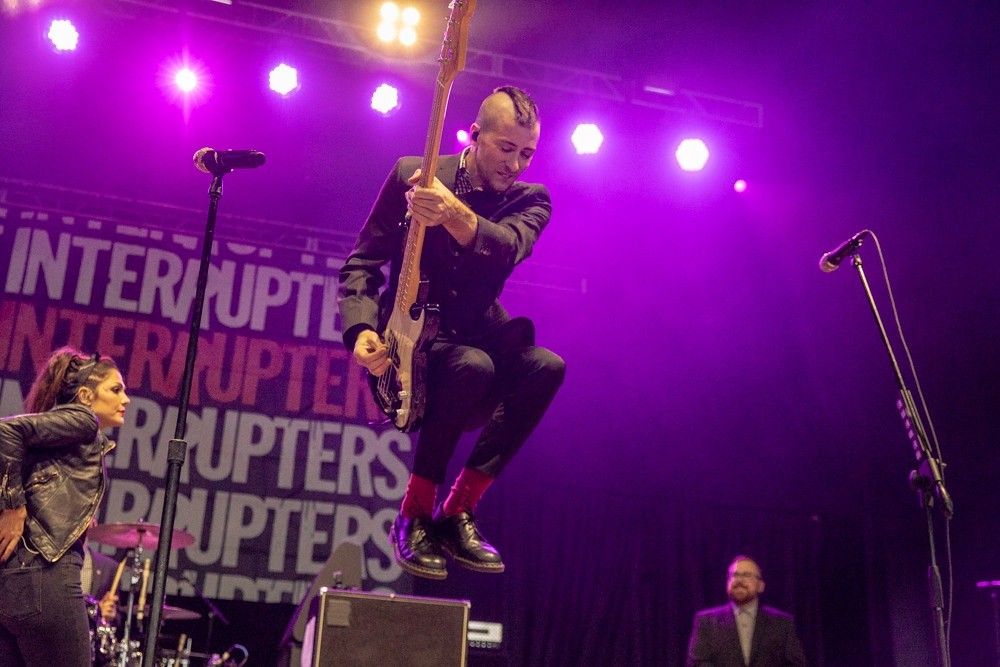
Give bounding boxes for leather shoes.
[431,501,505,574]
[389,512,448,580]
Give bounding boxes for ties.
[736,608,755,659]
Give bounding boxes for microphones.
[818,229,870,273]
[192,149,266,173]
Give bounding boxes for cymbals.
[117,604,202,621]
[84,520,196,550]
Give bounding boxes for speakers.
[313,588,475,667]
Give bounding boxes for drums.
[83,594,142,667]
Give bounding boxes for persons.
[1,348,130,667]
[337,85,563,581]
[80,515,123,644]
[686,554,800,666]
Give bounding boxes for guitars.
[365,0,477,435]
